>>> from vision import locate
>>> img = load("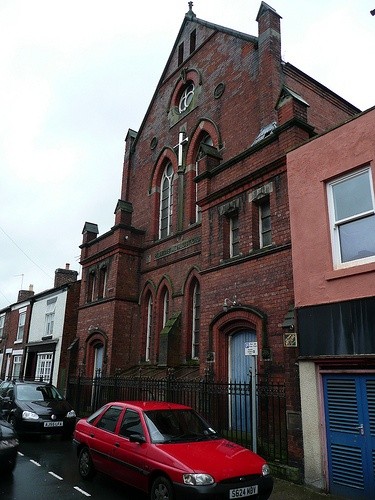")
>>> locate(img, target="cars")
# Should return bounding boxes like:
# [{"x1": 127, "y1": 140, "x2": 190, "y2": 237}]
[
  {"x1": 70, "y1": 400, "x2": 274, "y2": 500},
  {"x1": 0, "y1": 419, "x2": 18, "y2": 477},
  {"x1": 0, "y1": 379, "x2": 78, "y2": 442}
]
